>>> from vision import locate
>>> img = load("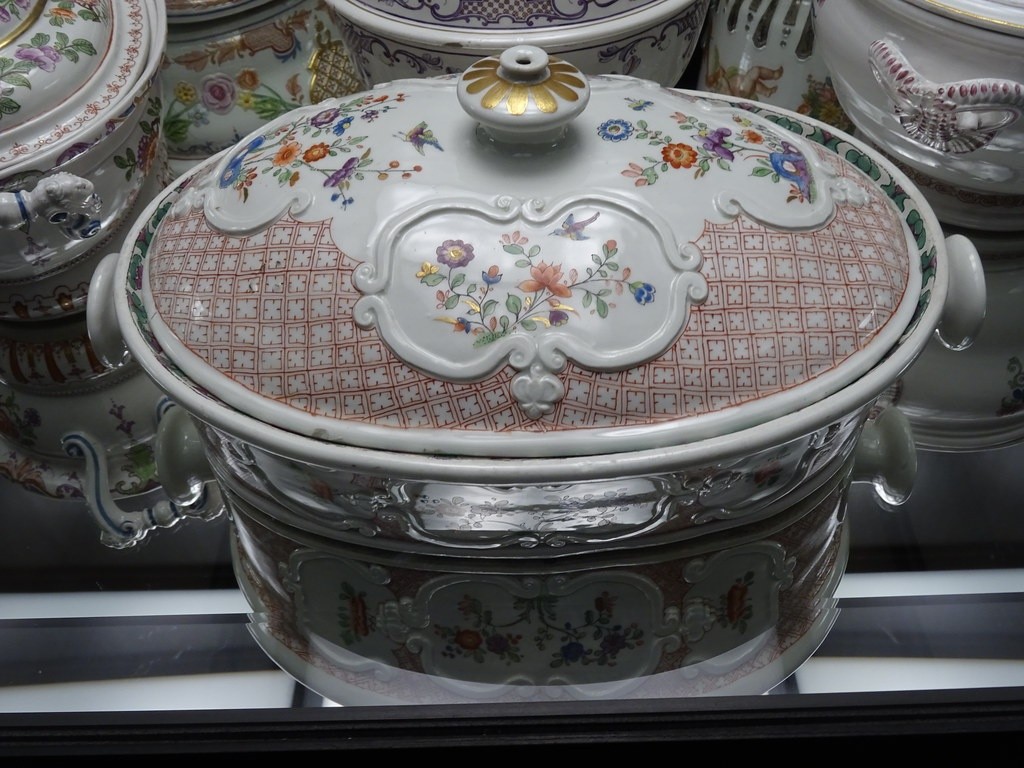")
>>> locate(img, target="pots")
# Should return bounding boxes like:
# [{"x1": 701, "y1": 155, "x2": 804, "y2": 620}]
[{"x1": 1, "y1": 0, "x2": 1022, "y2": 707}]
